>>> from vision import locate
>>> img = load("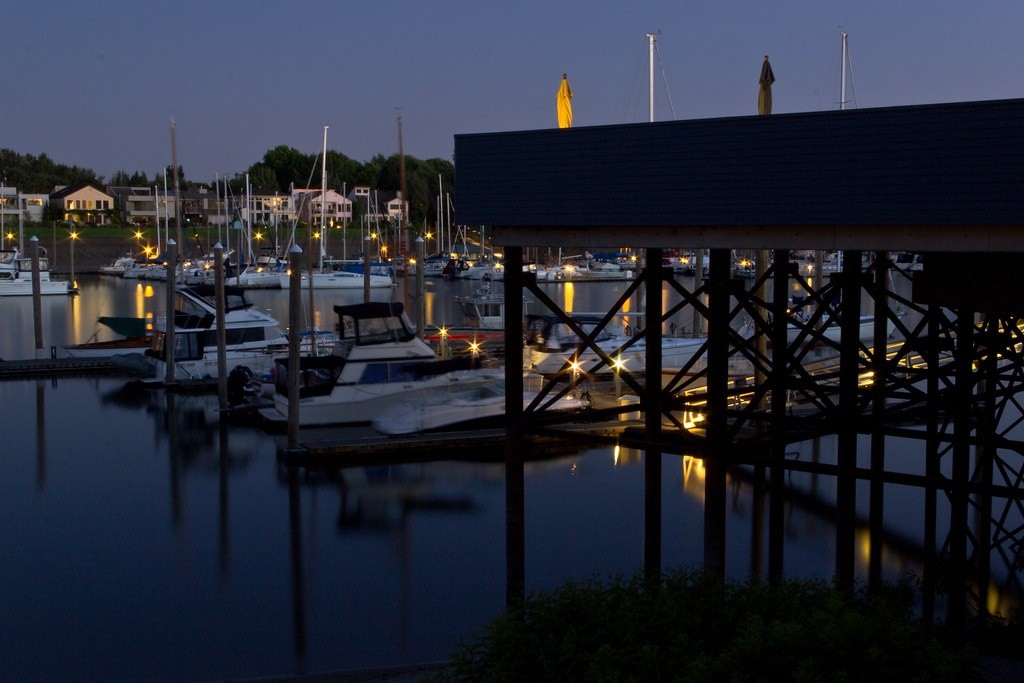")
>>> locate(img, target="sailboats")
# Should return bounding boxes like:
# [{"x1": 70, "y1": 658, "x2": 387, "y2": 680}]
[{"x1": 0, "y1": 116, "x2": 711, "y2": 438}]
